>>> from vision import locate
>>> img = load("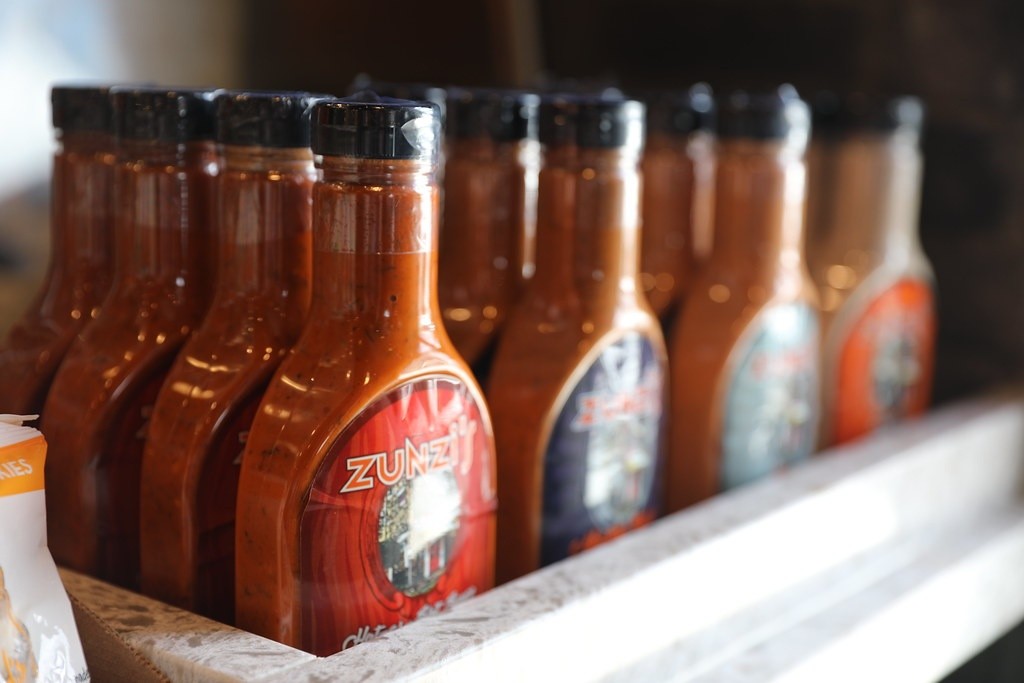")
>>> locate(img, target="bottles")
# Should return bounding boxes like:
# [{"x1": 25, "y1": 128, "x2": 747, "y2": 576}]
[{"x1": 0, "y1": 78, "x2": 942, "y2": 659}]
